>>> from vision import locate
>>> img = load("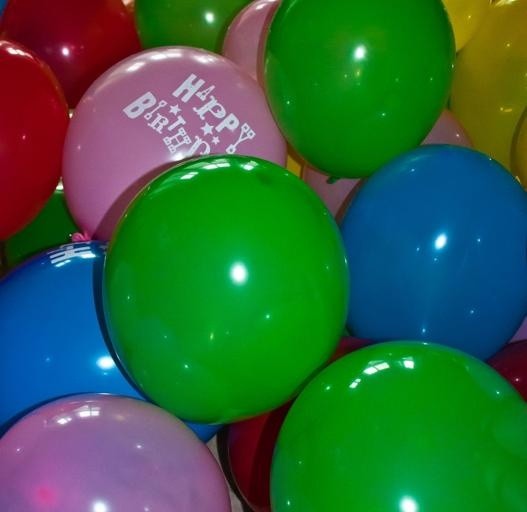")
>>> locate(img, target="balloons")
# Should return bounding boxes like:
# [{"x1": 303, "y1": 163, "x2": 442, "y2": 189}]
[{"x1": 1, "y1": 1, "x2": 527, "y2": 512}]
